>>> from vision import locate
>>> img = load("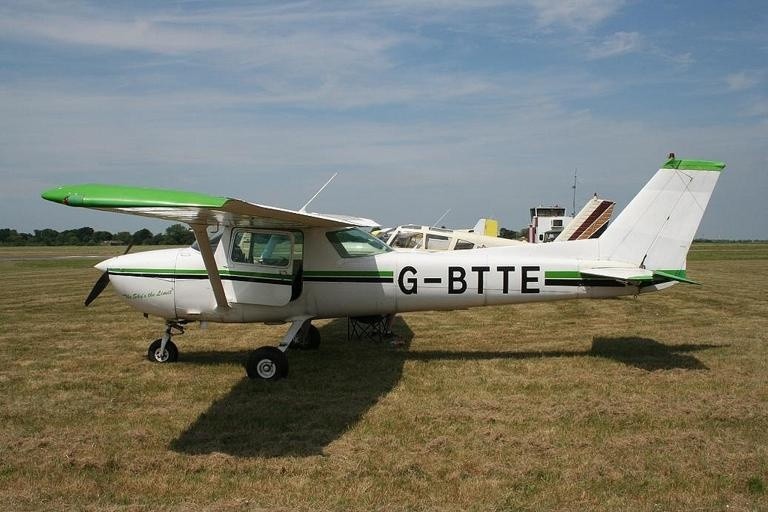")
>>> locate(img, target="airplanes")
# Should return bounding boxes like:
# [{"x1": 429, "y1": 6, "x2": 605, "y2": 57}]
[
  {"x1": 39, "y1": 153, "x2": 726, "y2": 385},
  {"x1": 340, "y1": 192, "x2": 617, "y2": 251}
]
